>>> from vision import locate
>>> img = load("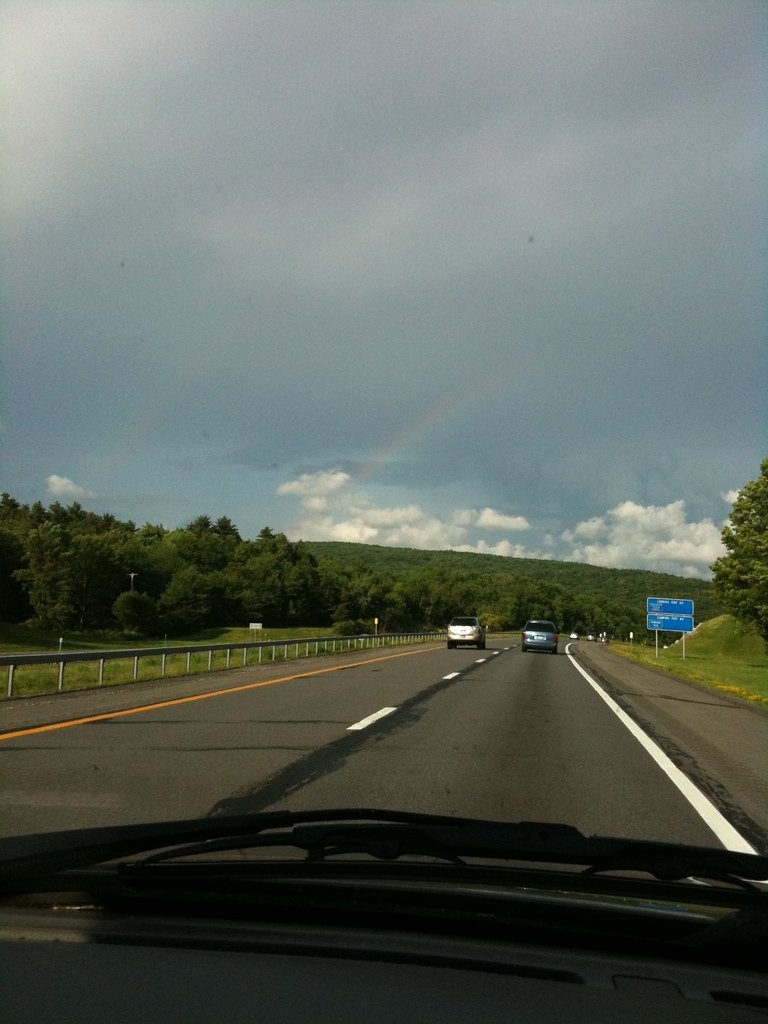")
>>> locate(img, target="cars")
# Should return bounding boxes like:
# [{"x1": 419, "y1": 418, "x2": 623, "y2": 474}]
[
  {"x1": 521, "y1": 620, "x2": 558, "y2": 655},
  {"x1": 570, "y1": 633, "x2": 580, "y2": 640},
  {"x1": 587, "y1": 633, "x2": 595, "y2": 641},
  {"x1": 447, "y1": 616, "x2": 488, "y2": 650}
]
